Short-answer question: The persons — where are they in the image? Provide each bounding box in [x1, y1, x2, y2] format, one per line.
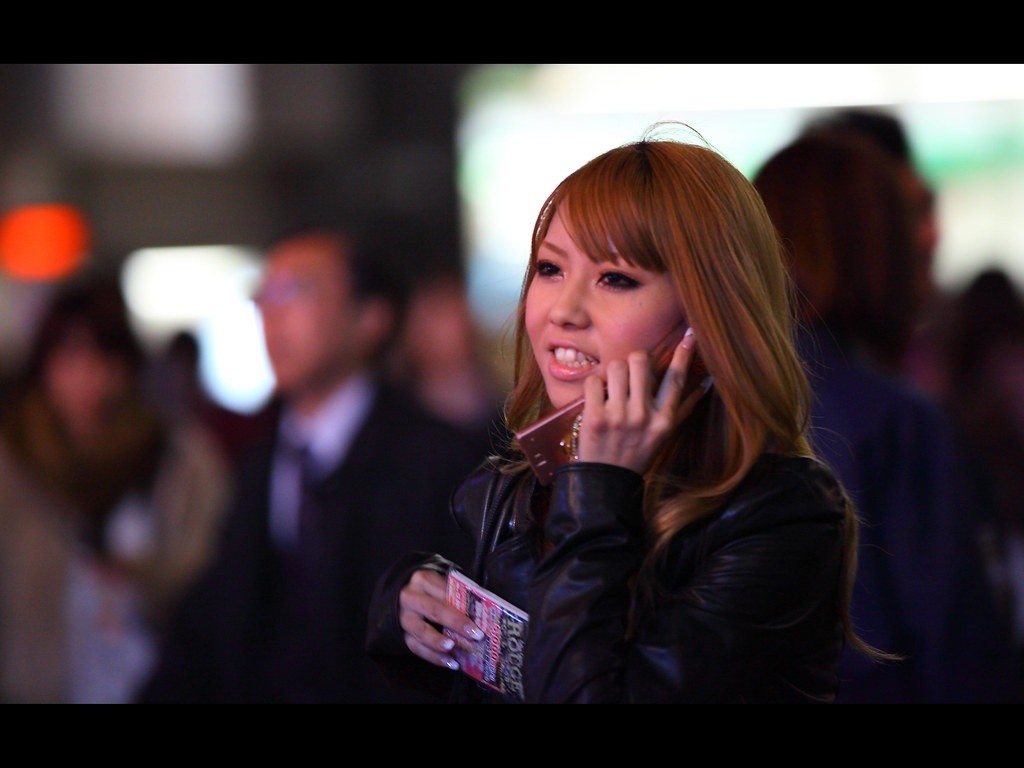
[368, 143, 904, 705]
[753, 107, 1024, 704]
[0, 206, 505, 706]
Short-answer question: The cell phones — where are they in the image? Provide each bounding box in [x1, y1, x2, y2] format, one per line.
[516, 325, 710, 486]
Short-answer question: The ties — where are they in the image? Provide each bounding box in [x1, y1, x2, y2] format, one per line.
[294, 447, 320, 538]
